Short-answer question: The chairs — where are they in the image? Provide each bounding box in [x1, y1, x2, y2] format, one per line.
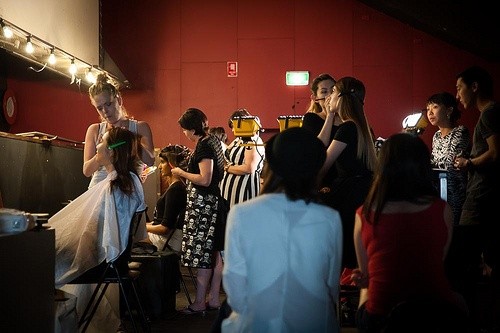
[64, 206, 150, 333]
[162, 212, 197, 306]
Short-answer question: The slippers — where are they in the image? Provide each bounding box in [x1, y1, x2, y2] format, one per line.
[206, 303, 220, 314]
[180, 306, 207, 318]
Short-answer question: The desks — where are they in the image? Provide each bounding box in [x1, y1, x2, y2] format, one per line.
[119, 251, 181, 330]
[0, 228, 55, 333]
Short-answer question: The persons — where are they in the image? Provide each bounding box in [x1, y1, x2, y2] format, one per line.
[316, 76, 380, 272]
[140, 145, 190, 252]
[218, 109, 267, 210]
[302, 72, 342, 207]
[426, 92, 472, 227]
[213, 127, 343, 333]
[169, 106, 228, 318]
[41, 127, 147, 333]
[82, 75, 155, 188]
[442, 65, 500, 333]
[351, 133, 454, 333]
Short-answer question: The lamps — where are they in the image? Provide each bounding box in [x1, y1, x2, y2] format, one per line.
[401, 112, 427, 135]
[233, 115, 264, 141]
[277, 115, 304, 132]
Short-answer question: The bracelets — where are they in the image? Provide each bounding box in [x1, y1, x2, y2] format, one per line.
[466, 159, 473, 168]
